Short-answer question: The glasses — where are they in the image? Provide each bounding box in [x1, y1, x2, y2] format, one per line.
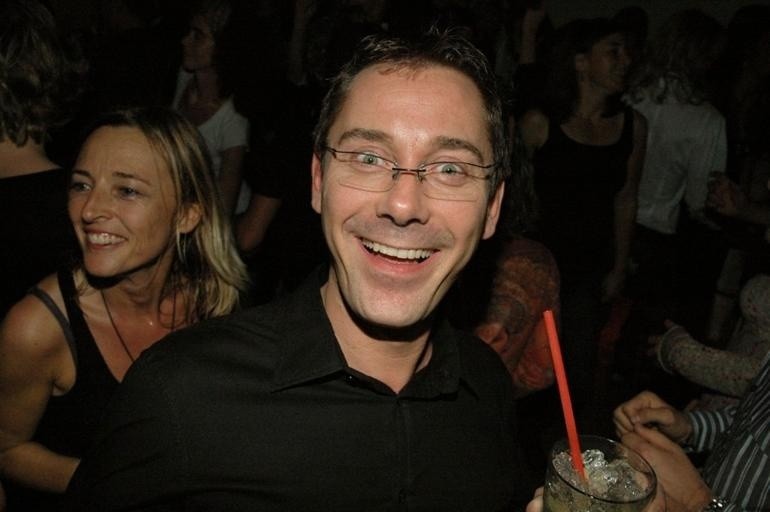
[326, 147, 495, 200]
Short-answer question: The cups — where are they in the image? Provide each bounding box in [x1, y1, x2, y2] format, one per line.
[541, 434, 657, 512]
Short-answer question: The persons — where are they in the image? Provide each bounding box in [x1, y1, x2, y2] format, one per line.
[449, 12, 770, 512]
[48, 25, 538, 511]
[3, 14, 332, 512]
